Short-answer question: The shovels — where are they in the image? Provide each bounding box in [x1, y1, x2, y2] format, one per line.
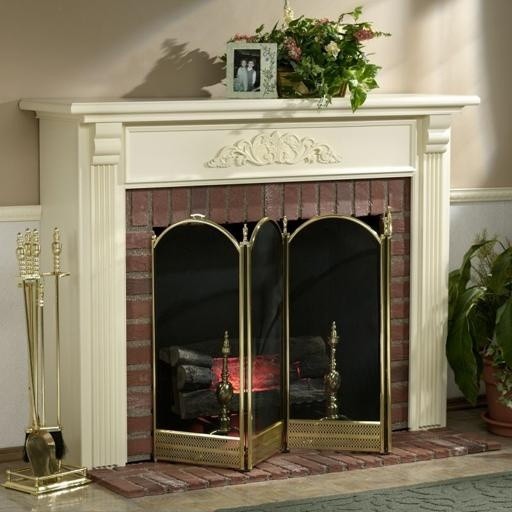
[16, 231, 59, 485]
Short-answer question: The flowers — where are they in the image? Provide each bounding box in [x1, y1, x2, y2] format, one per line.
[219, 0, 392, 116]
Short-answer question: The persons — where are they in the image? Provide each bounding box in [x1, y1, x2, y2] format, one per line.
[237, 59, 248, 92]
[248, 59, 257, 91]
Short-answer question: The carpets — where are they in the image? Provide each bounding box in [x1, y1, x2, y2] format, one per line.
[214, 467, 512, 512]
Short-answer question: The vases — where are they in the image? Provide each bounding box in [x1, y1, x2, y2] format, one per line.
[278, 65, 347, 99]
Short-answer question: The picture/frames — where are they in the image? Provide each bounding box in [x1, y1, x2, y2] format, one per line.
[226, 42, 278, 99]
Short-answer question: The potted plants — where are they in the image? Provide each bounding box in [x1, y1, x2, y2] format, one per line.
[445, 226, 512, 439]
